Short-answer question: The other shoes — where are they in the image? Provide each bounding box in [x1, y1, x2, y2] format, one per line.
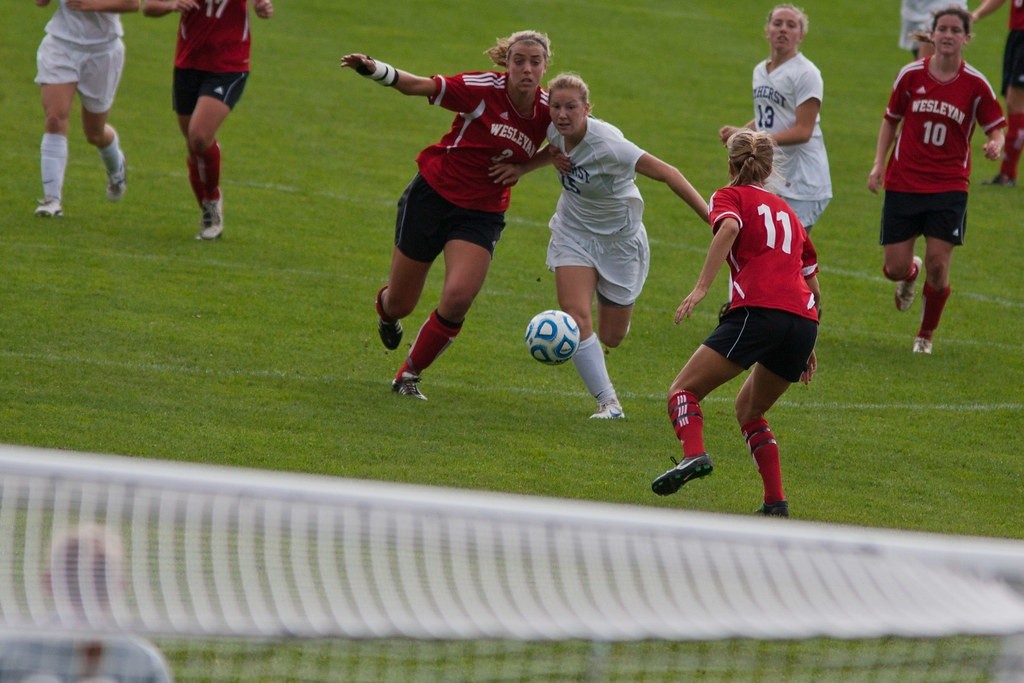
[981, 173, 1013, 186]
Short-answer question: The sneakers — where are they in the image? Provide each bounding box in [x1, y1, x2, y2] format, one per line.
[651, 453, 714, 496]
[105, 161, 126, 199]
[198, 189, 224, 240]
[753, 500, 789, 518]
[912, 335, 933, 355]
[894, 257, 922, 312]
[375, 286, 403, 349]
[589, 402, 624, 420]
[390, 377, 427, 402]
[33, 196, 63, 217]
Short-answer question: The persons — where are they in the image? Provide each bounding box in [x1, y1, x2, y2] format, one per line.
[717, 5, 834, 319]
[899, 0, 968, 62]
[491, 74, 714, 420]
[970, 0, 1024, 186]
[142, 0, 274, 239]
[0, 523, 173, 683]
[651, 133, 822, 522]
[33, 0, 141, 218]
[339, 30, 558, 397]
[868, 7, 1010, 353]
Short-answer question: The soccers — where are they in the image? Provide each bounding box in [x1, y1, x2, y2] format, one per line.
[524, 309, 580, 366]
